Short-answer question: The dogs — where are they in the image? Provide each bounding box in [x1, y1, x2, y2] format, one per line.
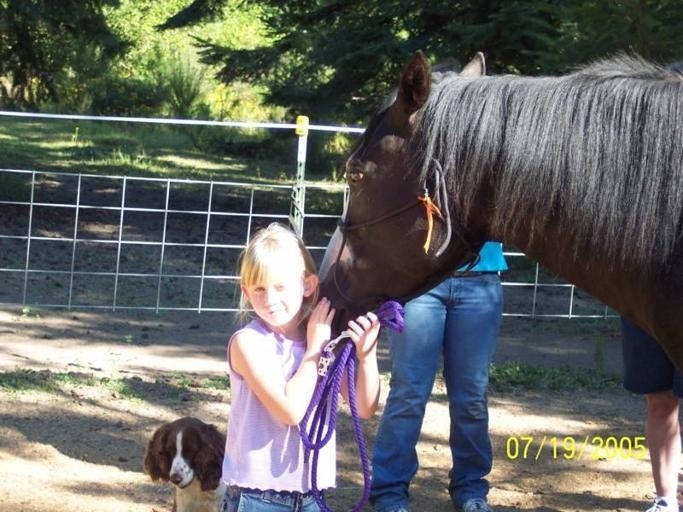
[141, 416, 227, 512]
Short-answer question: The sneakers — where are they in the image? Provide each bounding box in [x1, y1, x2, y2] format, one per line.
[461, 496, 495, 512]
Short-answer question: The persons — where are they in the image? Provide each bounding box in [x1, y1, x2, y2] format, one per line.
[618, 308, 683, 512]
[369, 240, 506, 512]
[219, 221, 381, 511]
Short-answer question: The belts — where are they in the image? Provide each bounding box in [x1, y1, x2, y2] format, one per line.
[452, 271, 501, 277]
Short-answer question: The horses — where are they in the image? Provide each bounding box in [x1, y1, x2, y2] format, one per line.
[304, 51, 683, 374]
